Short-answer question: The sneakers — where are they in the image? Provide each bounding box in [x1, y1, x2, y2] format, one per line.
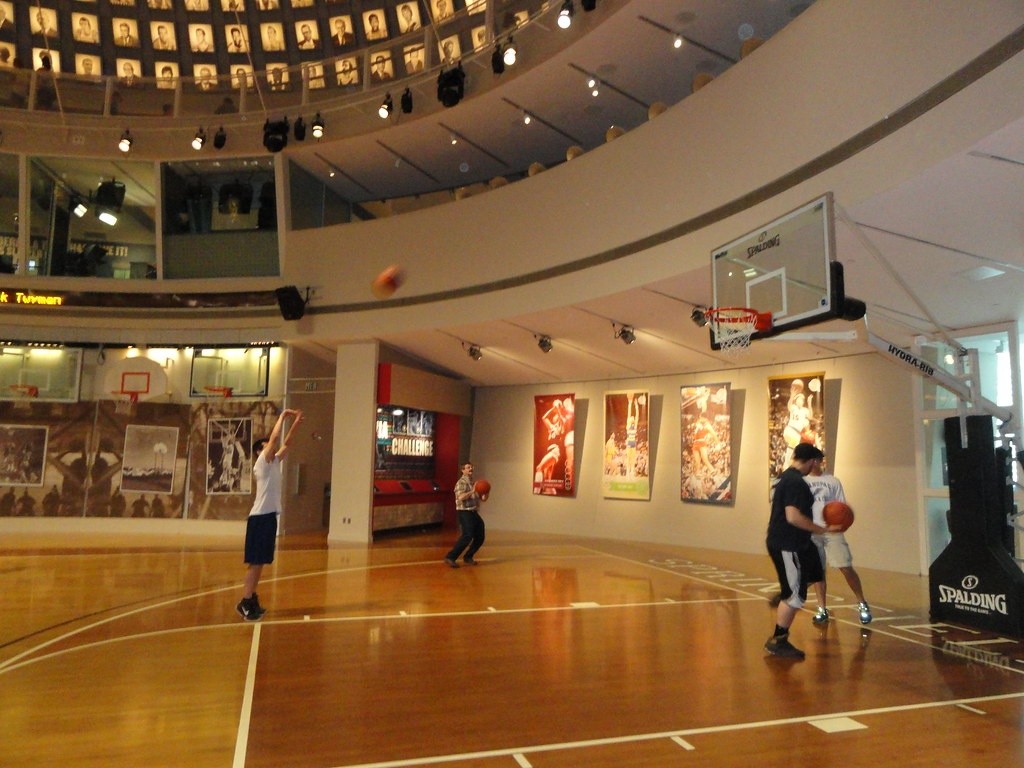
[858, 602, 871, 624]
[235, 599, 260, 621]
[444, 556, 458, 567]
[771, 594, 782, 606]
[251, 594, 266, 615]
[812, 607, 829, 624]
[765, 637, 805, 659]
[463, 556, 478, 566]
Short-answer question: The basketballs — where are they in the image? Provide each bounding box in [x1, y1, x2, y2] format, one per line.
[474, 479, 491, 494]
[822, 500, 854, 533]
[370, 264, 406, 299]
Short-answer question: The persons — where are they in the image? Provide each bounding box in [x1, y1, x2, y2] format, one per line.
[9, 57, 29, 108]
[163, 104, 170, 115]
[110, 91, 123, 115]
[37, 56, 54, 110]
[214, 97, 235, 114]
[765, 442, 843, 657]
[74, 0, 522, 91]
[0, 404, 270, 518]
[770, 386, 826, 479]
[0, 6, 12, 28]
[36, 11, 57, 37]
[605, 398, 648, 477]
[803, 449, 872, 623]
[536, 402, 574, 494]
[0, 47, 11, 66]
[237, 410, 305, 620]
[682, 389, 731, 500]
[445, 461, 489, 567]
[40, 52, 52, 63]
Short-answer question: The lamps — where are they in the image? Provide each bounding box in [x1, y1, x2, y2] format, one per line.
[70, 0, 596, 228]
[690, 305, 708, 327]
[461, 340, 483, 360]
[612, 322, 636, 345]
[534, 334, 553, 354]
